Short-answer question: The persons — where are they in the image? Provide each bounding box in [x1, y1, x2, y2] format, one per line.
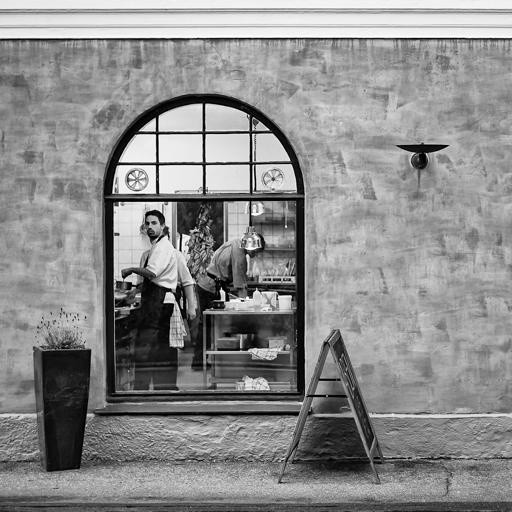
[138, 226, 197, 322]
[122, 210, 178, 390]
[191, 232, 265, 371]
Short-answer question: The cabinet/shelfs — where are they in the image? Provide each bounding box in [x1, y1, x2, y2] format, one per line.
[247, 199, 297, 290]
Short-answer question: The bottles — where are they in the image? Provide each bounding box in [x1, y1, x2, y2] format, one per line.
[253, 287, 262, 311]
[219, 287, 226, 302]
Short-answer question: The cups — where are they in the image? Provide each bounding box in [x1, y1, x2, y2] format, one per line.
[278, 295, 292, 311]
[262, 292, 277, 310]
[211, 300, 224, 309]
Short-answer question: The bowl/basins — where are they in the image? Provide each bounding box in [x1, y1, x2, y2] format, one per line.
[224, 332, 256, 350]
[256, 338, 287, 348]
[230, 299, 253, 311]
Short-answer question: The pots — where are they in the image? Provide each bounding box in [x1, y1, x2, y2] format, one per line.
[116, 281, 137, 290]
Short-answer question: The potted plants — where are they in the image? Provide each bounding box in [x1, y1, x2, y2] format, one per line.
[32, 307, 91, 471]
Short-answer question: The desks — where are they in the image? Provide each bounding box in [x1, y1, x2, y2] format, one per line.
[202, 308, 298, 393]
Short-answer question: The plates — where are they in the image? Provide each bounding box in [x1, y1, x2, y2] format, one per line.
[209, 309, 227, 311]
